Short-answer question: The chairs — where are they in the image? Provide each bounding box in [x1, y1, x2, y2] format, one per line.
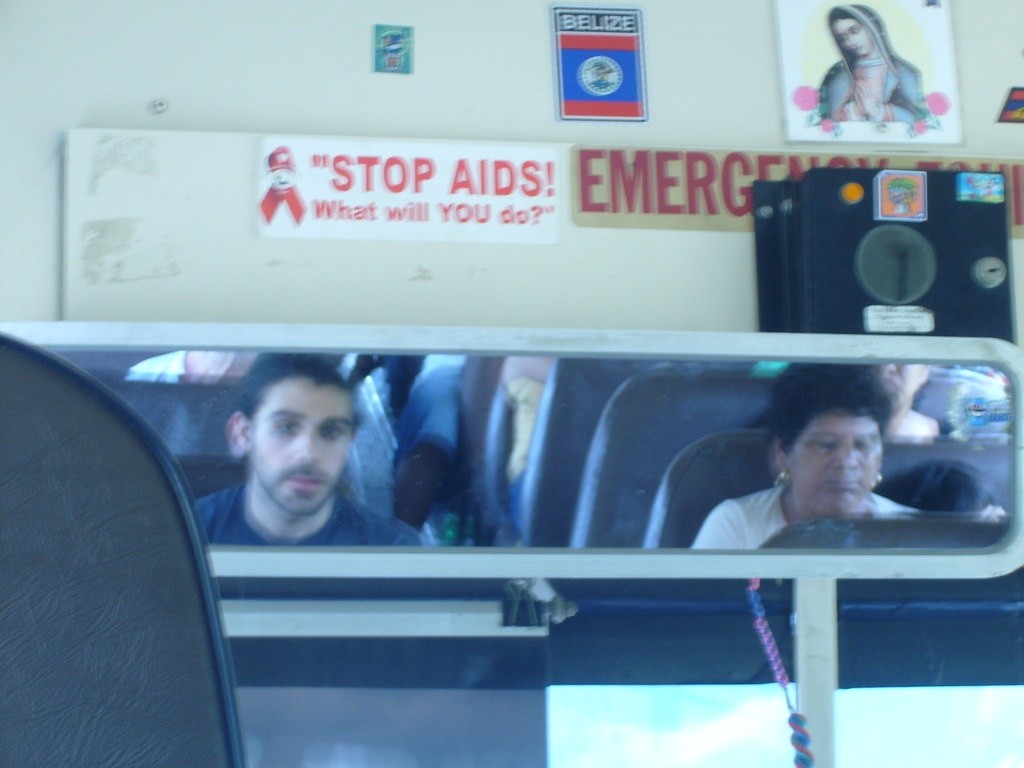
[112, 353, 1014, 547]
[0, 333, 245, 768]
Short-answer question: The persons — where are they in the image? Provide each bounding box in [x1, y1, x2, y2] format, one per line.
[691, 365, 968, 550]
[124, 349, 557, 545]
